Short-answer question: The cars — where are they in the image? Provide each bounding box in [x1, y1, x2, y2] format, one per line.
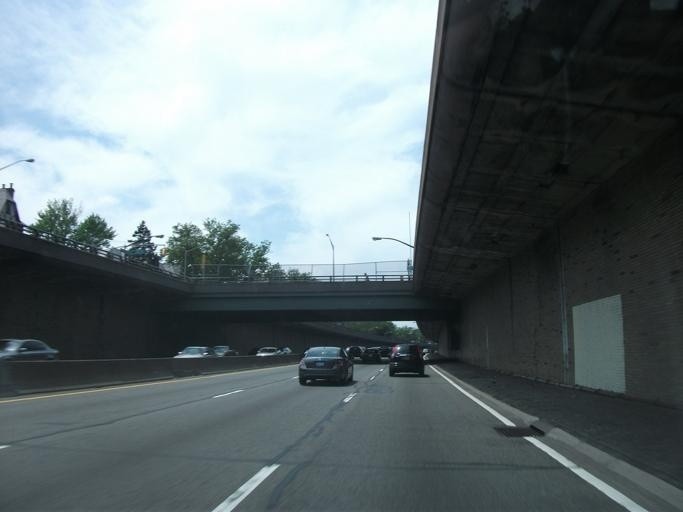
[346, 346, 390, 362]
[1, 338, 62, 360]
[174, 345, 238, 358]
[298, 347, 354, 385]
[390, 344, 425, 376]
[256, 347, 282, 356]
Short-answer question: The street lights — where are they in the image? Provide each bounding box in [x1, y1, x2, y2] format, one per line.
[373, 236, 413, 247]
[1, 158, 33, 172]
[124, 234, 163, 263]
[327, 234, 334, 282]
